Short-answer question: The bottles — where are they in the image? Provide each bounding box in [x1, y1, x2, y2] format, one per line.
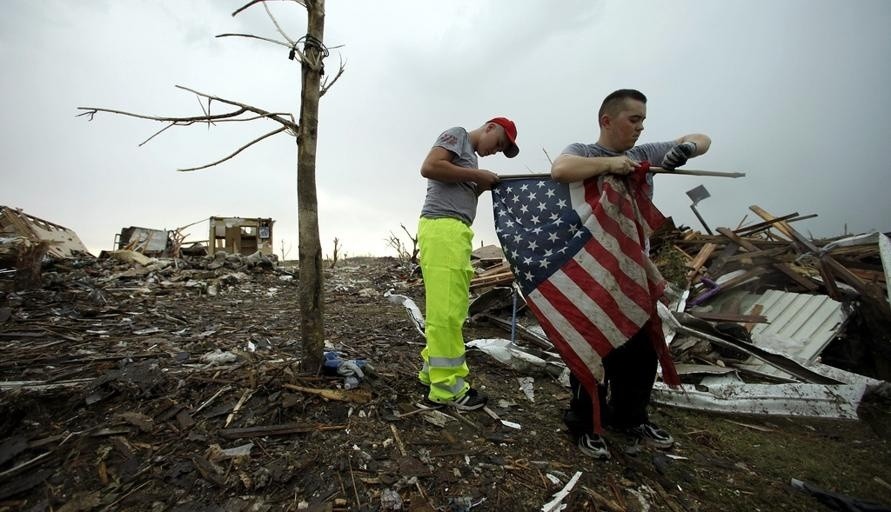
[344, 366, 366, 390]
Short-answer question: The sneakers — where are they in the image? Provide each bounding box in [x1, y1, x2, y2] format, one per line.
[431, 388, 488, 410]
[578, 430, 612, 461]
[633, 423, 674, 449]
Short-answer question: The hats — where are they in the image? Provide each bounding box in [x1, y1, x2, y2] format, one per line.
[487, 118, 519, 157]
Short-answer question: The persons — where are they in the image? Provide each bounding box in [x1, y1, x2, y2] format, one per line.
[550, 88, 713, 461]
[418, 117, 520, 412]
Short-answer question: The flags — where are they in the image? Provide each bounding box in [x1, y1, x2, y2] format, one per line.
[491, 163, 691, 434]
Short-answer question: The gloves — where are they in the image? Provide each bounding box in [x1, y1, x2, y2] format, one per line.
[661, 142, 697, 171]
